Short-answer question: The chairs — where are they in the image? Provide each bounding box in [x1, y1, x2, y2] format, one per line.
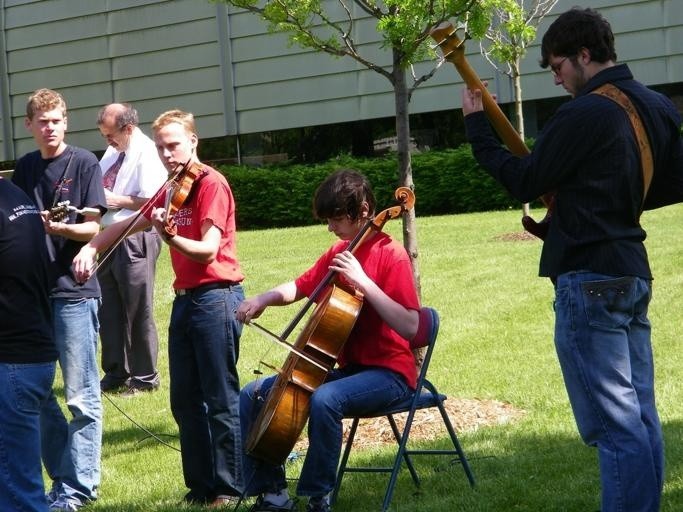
[330, 308, 475, 508]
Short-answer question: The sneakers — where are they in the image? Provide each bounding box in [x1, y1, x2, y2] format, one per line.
[47, 487, 60, 502]
[306, 496, 331, 512]
[250, 493, 299, 511]
[48, 497, 82, 511]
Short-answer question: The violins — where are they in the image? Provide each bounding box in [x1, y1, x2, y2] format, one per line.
[161, 158, 203, 241]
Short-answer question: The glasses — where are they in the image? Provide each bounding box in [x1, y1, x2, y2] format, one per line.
[100, 121, 132, 139]
[550, 56, 569, 76]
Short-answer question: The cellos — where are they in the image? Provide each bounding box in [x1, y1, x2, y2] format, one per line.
[243, 187, 415, 464]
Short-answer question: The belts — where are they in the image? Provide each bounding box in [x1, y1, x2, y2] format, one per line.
[174, 279, 240, 296]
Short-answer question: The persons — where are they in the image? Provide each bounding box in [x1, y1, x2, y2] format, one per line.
[461, 8, 683, 511]
[97, 102, 169, 394]
[235, 168, 422, 512]
[11, 89, 104, 511]
[0, 176, 58, 512]
[71, 109, 246, 507]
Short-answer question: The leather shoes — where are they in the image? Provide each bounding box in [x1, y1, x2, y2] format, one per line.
[179, 494, 209, 506]
[122, 384, 159, 394]
[100, 377, 127, 391]
[209, 496, 231, 509]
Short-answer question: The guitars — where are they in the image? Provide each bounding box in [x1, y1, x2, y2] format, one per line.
[427, 21, 561, 240]
[46, 203, 99, 222]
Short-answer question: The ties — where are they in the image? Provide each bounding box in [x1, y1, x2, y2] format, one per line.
[103, 152, 126, 191]
